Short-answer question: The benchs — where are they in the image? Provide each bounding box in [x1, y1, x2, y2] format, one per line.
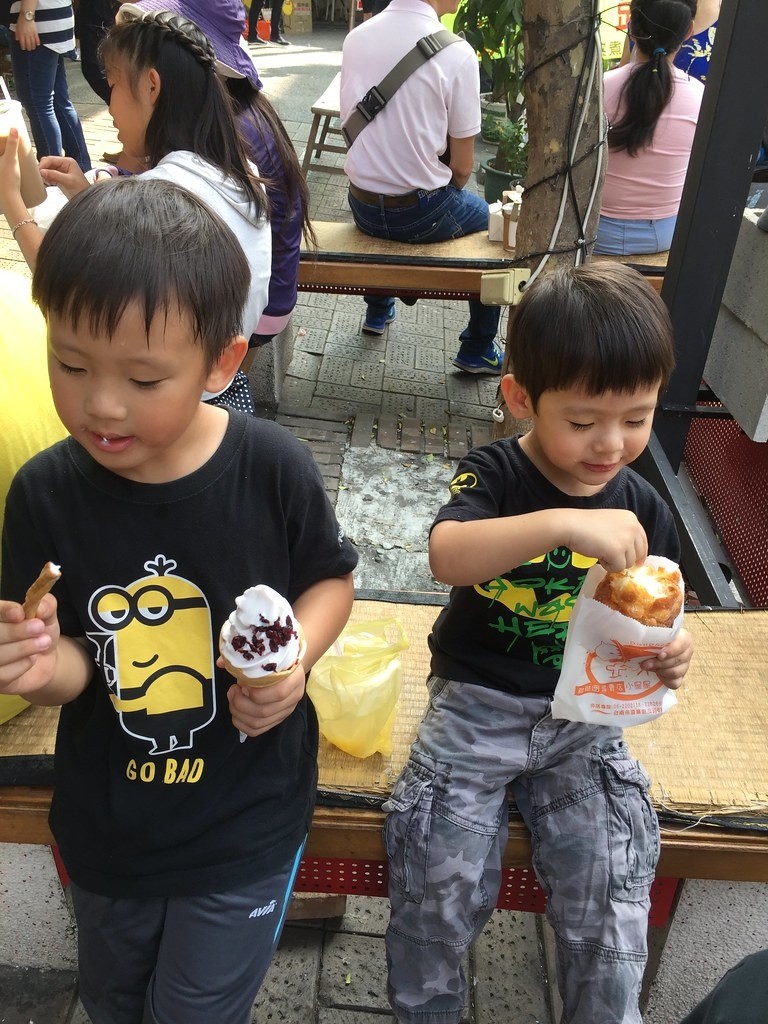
[296, 221, 671, 303]
[0, 588, 768, 883]
[301, 71, 349, 182]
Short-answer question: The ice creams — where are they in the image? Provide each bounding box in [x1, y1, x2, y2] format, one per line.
[217, 581, 307, 744]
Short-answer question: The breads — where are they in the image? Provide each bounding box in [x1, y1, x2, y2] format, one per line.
[596, 565, 684, 628]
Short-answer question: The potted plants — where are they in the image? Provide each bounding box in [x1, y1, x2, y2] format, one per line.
[453, 0, 528, 204]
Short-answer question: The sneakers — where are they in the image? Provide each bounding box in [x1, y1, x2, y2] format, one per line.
[362, 304, 396, 335]
[452, 342, 506, 375]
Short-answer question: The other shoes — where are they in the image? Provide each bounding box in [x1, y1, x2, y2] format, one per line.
[269, 37, 290, 45]
[248, 36, 268, 45]
[75, 47, 81, 61]
[103, 143, 123, 163]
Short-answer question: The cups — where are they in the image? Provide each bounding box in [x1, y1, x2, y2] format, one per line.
[502, 204, 522, 252]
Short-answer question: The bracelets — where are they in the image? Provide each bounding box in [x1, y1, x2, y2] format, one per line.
[12, 219, 38, 240]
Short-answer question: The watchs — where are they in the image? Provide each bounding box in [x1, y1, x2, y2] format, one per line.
[19, 10, 36, 21]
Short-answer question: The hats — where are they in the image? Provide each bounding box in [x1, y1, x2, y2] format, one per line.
[118, 0, 264, 92]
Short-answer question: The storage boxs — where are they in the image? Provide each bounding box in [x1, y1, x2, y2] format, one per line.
[242, 20, 271, 41]
[281, 0, 312, 35]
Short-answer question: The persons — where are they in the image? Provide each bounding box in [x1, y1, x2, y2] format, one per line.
[339, 0, 505, 373]
[593, 1, 704, 258]
[0, 183, 356, 1023]
[0, 12, 273, 402]
[246, 1, 293, 47]
[0, 0, 91, 186]
[384, 260, 695, 1022]
[94, 1, 322, 349]
[619, 1, 720, 83]
